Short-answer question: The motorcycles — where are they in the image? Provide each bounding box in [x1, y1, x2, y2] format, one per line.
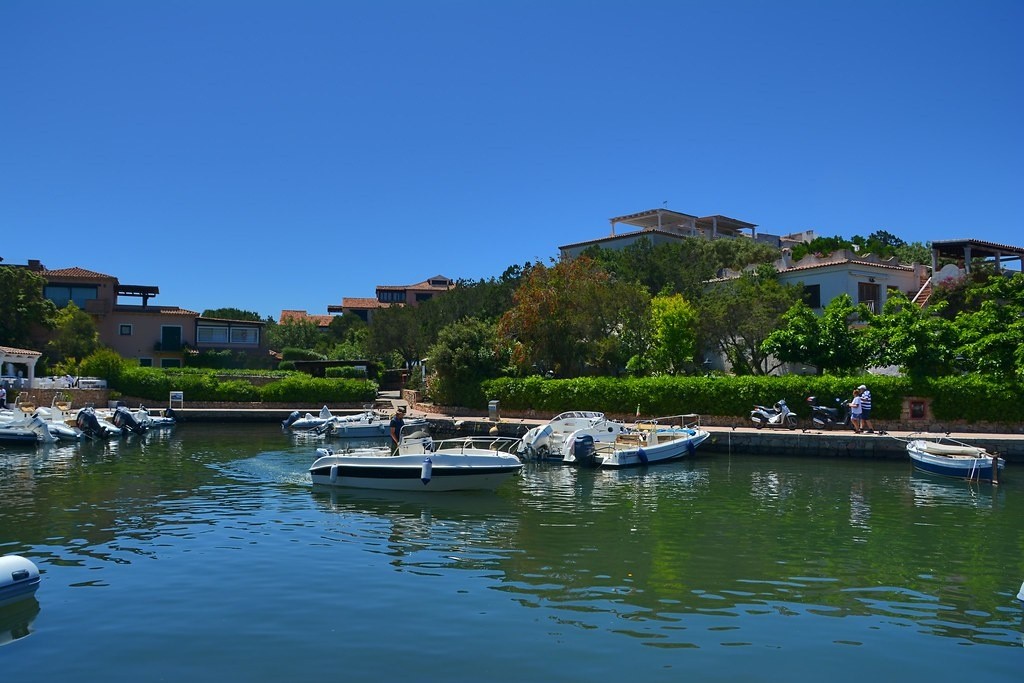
[807, 395, 861, 430]
[750, 397, 798, 431]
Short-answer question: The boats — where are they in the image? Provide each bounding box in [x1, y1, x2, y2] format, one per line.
[308, 431, 524, 490]
[574, 412, 710, 468]
[291, 404, 432, 439]
[517, 411, 611, 463]
[907, 439, 1009, 480]
[0, 391, 176, 442]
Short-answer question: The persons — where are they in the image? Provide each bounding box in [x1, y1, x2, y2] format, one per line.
[857, 385, 874, 433]
[0, 385, 10, 409]
[848, 390, 862, 434]
[390, 407, 406, 456]
[64, 374, 75, 388]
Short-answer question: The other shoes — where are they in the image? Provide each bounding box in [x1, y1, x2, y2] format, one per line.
[853, 431, 863, 434]
[865, 430, 873, 434]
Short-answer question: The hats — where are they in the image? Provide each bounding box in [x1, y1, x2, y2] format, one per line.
[858, 385, 866, 390]
